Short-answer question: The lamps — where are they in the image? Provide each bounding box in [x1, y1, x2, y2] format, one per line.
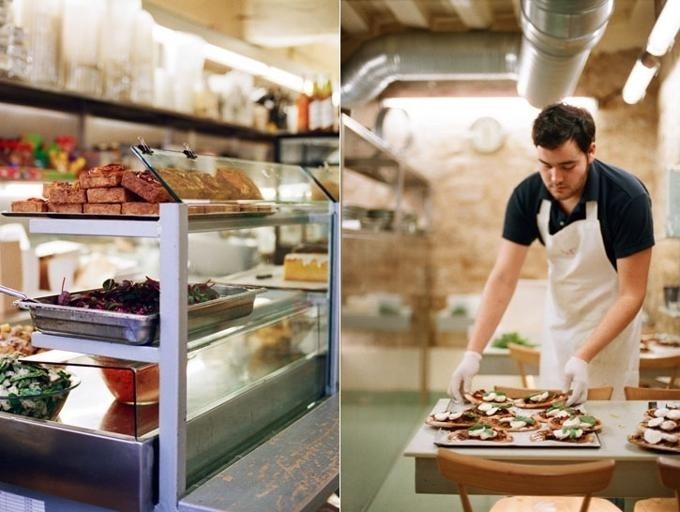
[622, 0, 679, 106]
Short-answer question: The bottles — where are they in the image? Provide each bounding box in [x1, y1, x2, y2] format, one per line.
[253, 80, 335, 139]
[2, 136, 123, 180]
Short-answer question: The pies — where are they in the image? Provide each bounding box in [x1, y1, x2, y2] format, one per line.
[425, 390, 680, 453]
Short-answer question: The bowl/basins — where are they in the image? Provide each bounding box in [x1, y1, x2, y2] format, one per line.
[1, 375, 81, 423]
[88, 354, 160, 407]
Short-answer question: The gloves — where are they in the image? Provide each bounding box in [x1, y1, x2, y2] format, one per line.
[448, 350, 483, 401]
[560, 355, 591, 408]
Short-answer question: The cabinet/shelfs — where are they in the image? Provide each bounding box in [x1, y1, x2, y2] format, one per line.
[0, 197, 339, 512]
[269, 128, 340, 260]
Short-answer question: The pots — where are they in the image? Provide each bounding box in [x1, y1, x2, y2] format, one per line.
[340, 204, 415, 232]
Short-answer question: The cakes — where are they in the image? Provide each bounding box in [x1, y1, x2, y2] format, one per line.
[283, 243, 329, 282]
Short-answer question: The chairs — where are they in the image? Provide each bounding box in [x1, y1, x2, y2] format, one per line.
[631, 456, 680, 512]
[435, 445, 624, 512]
[490, 331, 680, 401]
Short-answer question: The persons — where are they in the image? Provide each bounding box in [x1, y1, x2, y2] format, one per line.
[445, 102, 658, 410]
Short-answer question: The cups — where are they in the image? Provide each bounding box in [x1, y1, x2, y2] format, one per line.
[0, 3, 160, 109]
[663, 287, 680, 312]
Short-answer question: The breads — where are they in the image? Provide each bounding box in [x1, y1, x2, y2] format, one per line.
[12, 164, 271, 217]
